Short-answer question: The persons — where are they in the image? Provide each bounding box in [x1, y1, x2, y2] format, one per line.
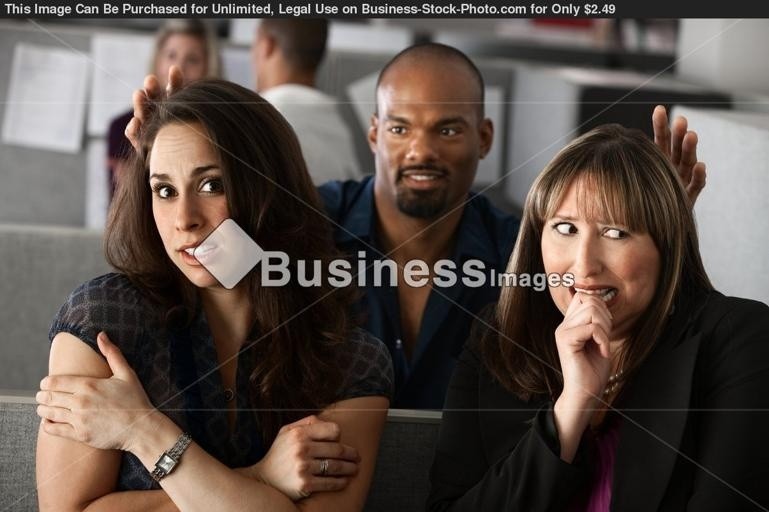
[36, 18, 768, 512]
